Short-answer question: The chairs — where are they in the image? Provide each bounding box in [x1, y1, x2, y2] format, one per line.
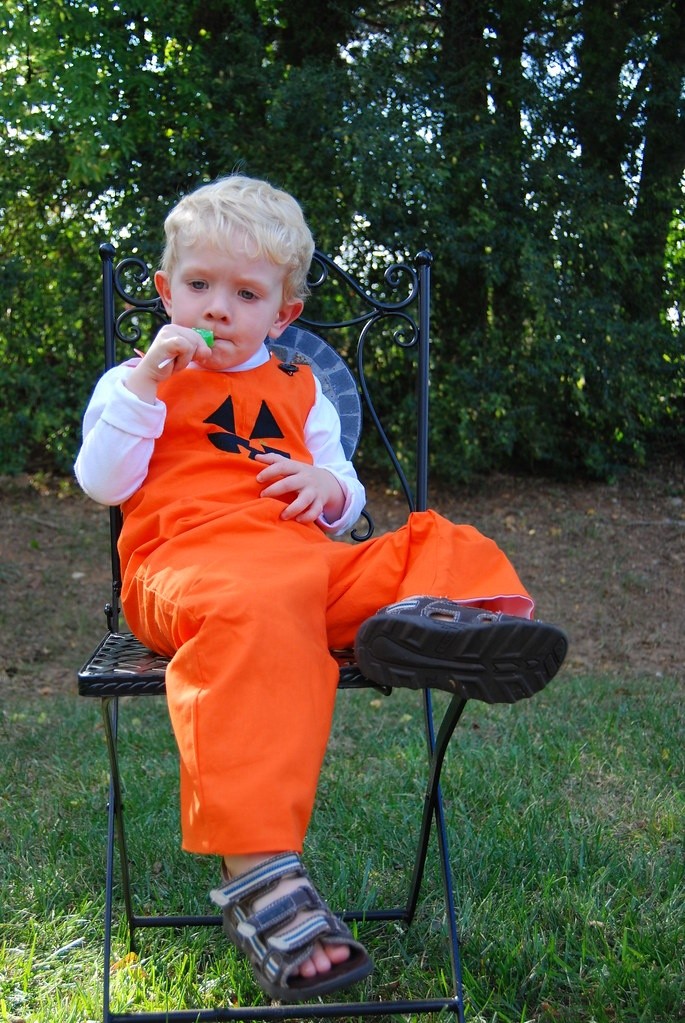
[78, 243, 468, 1023]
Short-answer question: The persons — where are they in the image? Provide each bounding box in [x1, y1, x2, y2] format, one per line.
[65, 176, 572, 1006]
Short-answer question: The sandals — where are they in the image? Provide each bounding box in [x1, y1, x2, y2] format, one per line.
[209, 849, 374, 1003]
[354, 594, 568, 704]
